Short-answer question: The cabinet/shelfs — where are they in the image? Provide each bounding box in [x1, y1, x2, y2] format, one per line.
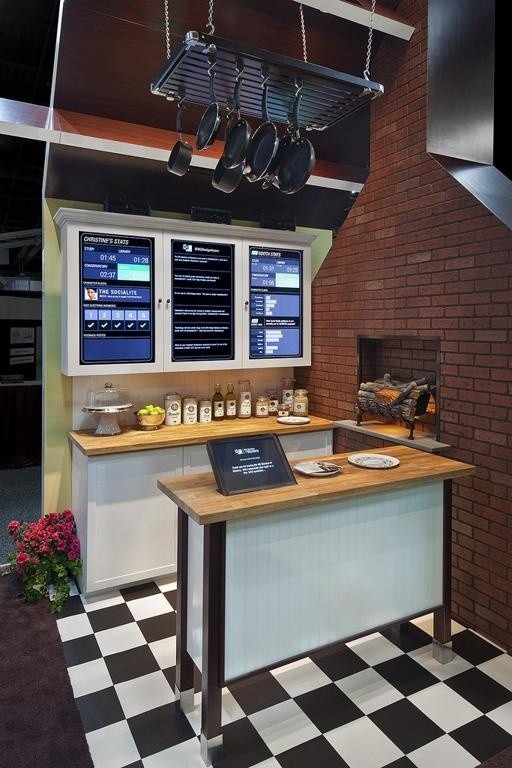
[68, 431, 214, 596]
[243, 227, 317, 368]
[54, 208, 243, 377]
[190, 423, 335, 475]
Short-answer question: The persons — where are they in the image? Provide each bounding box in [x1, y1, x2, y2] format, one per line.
[86, 287, 97, 301]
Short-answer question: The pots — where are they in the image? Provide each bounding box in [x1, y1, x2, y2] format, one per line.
[167, 51, 315, 198]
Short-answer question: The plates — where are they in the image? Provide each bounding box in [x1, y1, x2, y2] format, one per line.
[347, 453, 400, 470]
[296, 460, 342, 478]
[275, 415, 311, 424]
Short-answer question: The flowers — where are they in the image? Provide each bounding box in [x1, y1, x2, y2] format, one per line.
[7, 509, 81, 615]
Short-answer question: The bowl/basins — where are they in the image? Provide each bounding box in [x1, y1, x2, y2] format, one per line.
[133, 411, 165, 430]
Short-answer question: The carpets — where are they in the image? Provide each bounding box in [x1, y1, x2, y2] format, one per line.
[0, 596, 94, 767]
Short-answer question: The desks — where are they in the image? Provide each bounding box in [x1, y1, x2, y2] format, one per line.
[157, 445, 477, 766]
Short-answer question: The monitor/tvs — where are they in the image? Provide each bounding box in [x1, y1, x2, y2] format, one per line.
[205, 432, 297, 497]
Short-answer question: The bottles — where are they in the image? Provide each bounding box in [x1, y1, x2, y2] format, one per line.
[237, 378, 252, 420]
[227, 383, 238, 420]
[255, 375, 308, 418]
[212, 383, 225, 420]
